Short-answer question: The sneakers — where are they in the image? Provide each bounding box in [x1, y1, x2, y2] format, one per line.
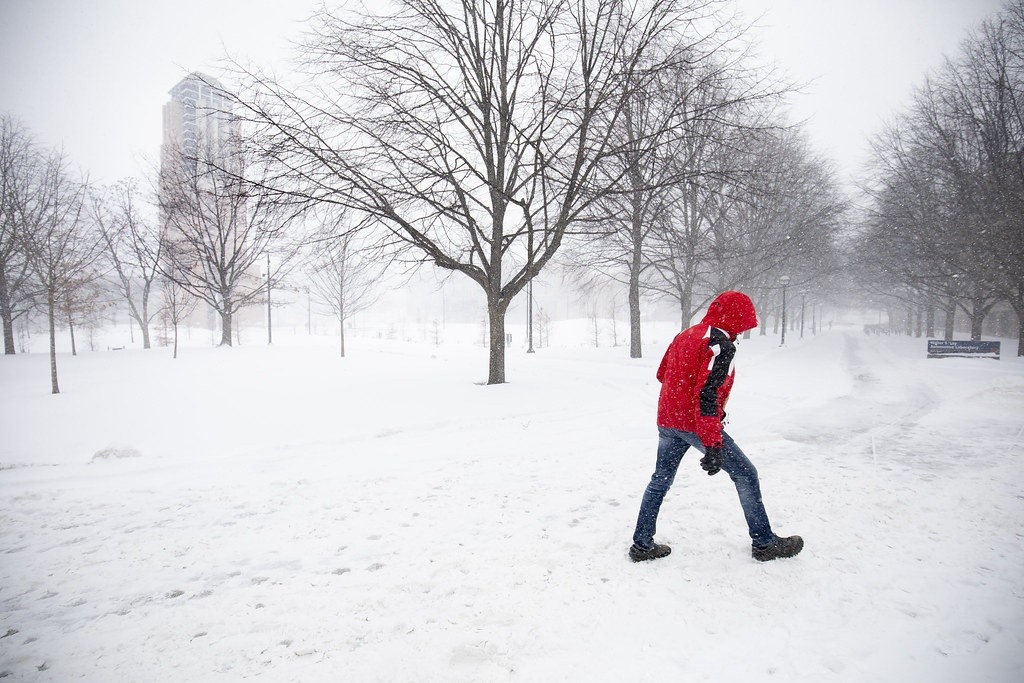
[752, 535, 803, 562]
[630, 545, 670, 561]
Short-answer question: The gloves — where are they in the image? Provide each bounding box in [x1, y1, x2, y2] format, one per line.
[700, 447, 722, 475]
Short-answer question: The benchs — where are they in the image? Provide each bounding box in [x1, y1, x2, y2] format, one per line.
[926, 340, 1001, 359]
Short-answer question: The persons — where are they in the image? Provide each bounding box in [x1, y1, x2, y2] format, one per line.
[629, 290, 804, 560]
